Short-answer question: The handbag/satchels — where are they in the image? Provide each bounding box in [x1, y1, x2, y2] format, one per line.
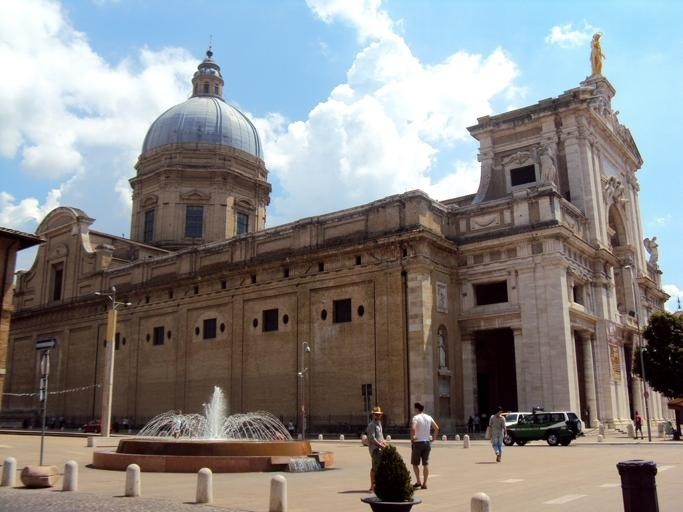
[484, 426, 492, 440]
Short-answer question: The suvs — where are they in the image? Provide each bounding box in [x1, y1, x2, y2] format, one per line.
[503, 408, 581, 445]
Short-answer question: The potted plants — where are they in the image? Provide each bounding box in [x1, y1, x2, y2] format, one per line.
[361, 441, 424, 511]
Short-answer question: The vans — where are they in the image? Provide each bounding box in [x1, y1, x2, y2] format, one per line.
[500, 412, 532, 427]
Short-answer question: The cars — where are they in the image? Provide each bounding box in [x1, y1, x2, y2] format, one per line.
[81, 420, 113, 433]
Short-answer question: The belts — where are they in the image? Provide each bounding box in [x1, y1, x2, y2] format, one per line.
[413, 441, 430, 444]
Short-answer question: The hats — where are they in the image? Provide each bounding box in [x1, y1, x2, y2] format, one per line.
[371, 406, 383, 415]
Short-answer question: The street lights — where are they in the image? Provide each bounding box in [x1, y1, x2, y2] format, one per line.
[623, 265, 652, 442]
[94, 286, 133, 436]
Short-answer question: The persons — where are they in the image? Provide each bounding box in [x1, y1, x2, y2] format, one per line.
[28, 416, 66, 431]
[109, 415, 137, 434]
[365, 405, 390, 490]
[437, 328, 449, 369]
[408, 401, 439, 489]
[467, 415, 473, 433]
[603, 175, 629, 207]
[648, 235, 660, 263]
[473, 413, 480, 433]
[589, 32, 607, 74]
[287, 418, 295, 438]
[480, 414, 487, 431]
[489, 406, 507, 461]
[633, 410, 643, 439]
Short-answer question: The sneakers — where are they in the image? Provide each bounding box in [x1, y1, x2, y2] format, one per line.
[421, 485, 427, 489]
[413, 482, 421, 488]
[496, 454, 501, 462]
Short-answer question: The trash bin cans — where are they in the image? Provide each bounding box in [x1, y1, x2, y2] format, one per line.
[617, 460, 659, 512]
